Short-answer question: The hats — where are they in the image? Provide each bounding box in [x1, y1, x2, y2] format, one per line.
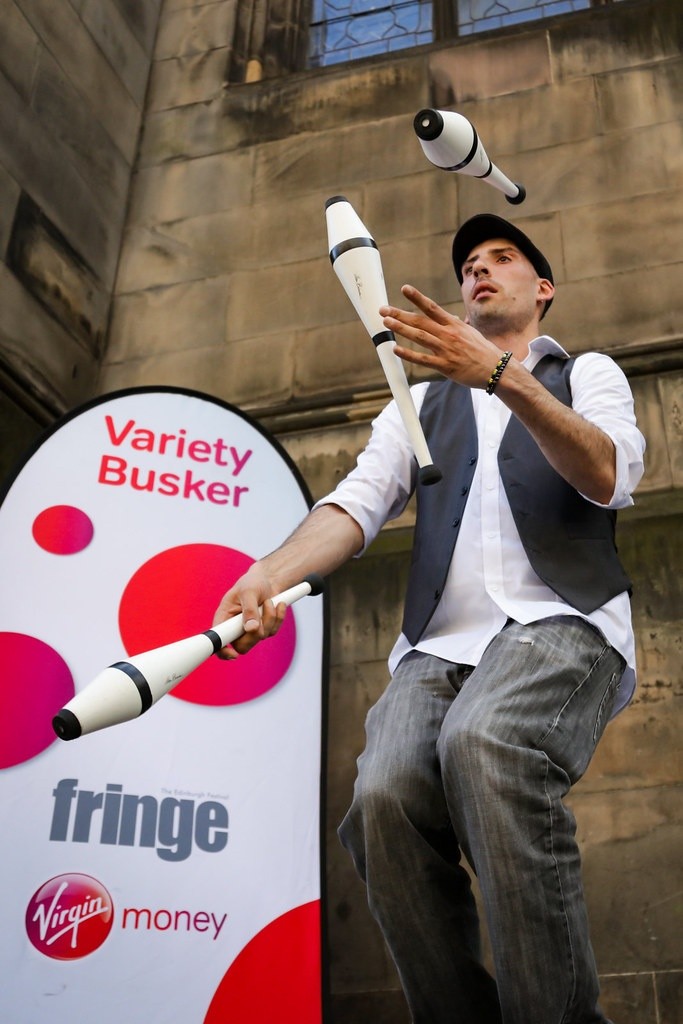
[452, 213, 555, 321]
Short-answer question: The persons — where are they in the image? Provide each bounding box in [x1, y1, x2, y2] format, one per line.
[212, 213, 646, 1024]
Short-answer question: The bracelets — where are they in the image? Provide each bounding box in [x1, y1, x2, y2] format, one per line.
[486, 350, 514, 396]
